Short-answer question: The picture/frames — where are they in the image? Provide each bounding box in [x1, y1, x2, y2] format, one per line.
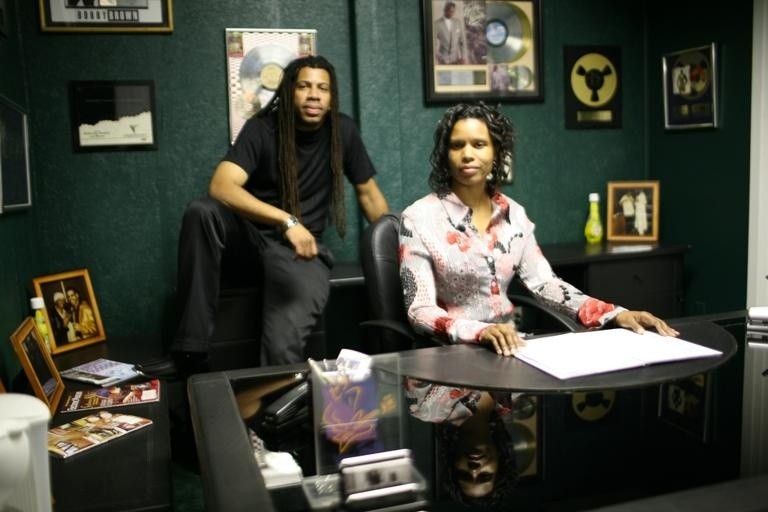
[12, 318, 65, 415]
[662, 44, 717, 129]
[0, 114, 34, 214]
[418, 0, 545, 107]
[71, 81, 156, 149]
[32, 270, 105, 354]
[41, 1, 176, 32]
[606, 181, 659, 242]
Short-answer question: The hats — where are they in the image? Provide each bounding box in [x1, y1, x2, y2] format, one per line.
[53, 292, 64, 302]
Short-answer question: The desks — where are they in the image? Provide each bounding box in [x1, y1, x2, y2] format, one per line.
[10, 334, 173, 512]
[186, 314, 768, 512]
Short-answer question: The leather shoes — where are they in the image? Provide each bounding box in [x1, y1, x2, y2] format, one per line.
[137, 351, 219, 380]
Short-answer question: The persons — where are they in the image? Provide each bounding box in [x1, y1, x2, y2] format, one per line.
[676, 69, 688, 94]
[435, 2, 468, 65]
[50, 292, 80, 347]
[402, 373, 517, 509]
[67, 288, 98, 339]
[634, 189, 647, 237]
[166, 56, 391, 365]
[396, 104, 680, 359]
[491, 65, 509, 92]
[620, 189, 635, 235]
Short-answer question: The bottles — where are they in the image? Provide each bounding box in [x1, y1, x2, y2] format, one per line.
[584, 193, 604, 243]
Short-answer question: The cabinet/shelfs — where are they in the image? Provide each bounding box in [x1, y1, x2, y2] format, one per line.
[323, 240, 688, 355]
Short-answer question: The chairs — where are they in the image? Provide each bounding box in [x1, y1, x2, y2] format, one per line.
[350, 212, 589, 352]
